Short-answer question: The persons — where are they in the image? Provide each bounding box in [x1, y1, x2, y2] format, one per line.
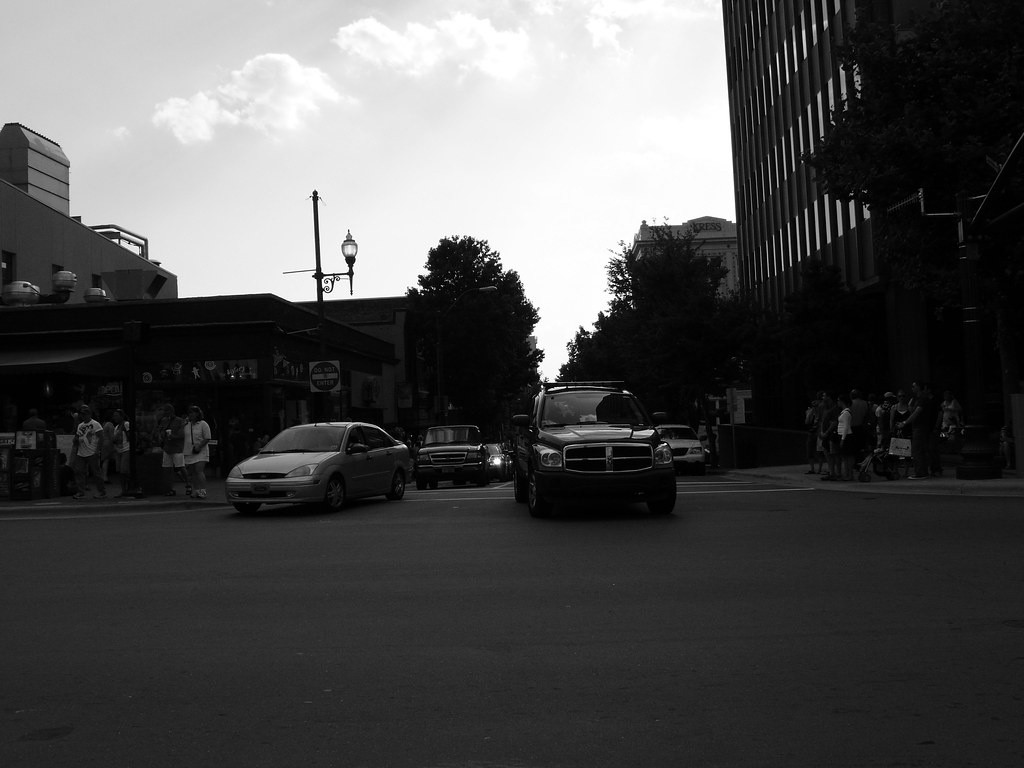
[2, 402, 213, 501]
[807, 382, 962, 480]
[224, 415, 423, 473]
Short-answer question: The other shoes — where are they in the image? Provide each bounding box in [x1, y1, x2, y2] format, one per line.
[804, 470, 816, 474]
[113, 492, 133, 498]
[93, 492, 107, 498]
[820, 474, 837, 480]
[73, 493, 85, 499]
[842, 475, 854, 481]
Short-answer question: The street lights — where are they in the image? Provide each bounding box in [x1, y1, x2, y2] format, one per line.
[283, 191, 358, 422]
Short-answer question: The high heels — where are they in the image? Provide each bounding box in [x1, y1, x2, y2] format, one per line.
[165, 490, 177, 496]
[185, 485, 192, 495]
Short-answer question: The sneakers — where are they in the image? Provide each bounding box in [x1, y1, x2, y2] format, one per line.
[908, 473, 928, 480]
[190, 489, 208, 498]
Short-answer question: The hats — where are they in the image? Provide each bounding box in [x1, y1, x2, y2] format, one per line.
[884, 392, 896, 397]
[80, 408, 92, 415]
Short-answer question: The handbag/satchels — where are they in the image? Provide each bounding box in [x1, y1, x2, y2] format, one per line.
[191, 422, 199, 454]
[160, 420, 184, 455]
[888, 430, 911, 457]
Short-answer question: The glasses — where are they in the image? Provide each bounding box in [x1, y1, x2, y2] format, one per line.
[898, 395, 905, 398]
[912, 385, 915, 388]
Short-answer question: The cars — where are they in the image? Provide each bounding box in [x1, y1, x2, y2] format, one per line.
[510, 381, 676, 518]
[416, 425, 705, 490]
[226, 422, 410, 512]
